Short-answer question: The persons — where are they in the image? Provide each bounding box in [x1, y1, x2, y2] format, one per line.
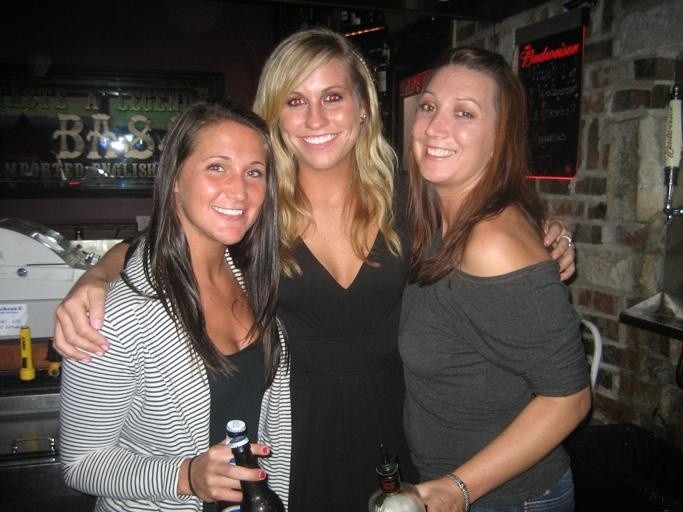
[52, 28, 576, 512]
[58, 104, 291, 511]
[398, 45, 593, 511]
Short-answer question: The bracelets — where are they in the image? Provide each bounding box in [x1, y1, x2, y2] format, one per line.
[446, 472, 470, 512]
[187, 455, 199, 497]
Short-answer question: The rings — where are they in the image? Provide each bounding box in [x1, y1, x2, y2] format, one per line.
[562, 235, 573, 247]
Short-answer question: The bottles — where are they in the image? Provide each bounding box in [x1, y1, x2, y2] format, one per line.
[208, 421, 287, 512]
[365, 442, 425, 512]
